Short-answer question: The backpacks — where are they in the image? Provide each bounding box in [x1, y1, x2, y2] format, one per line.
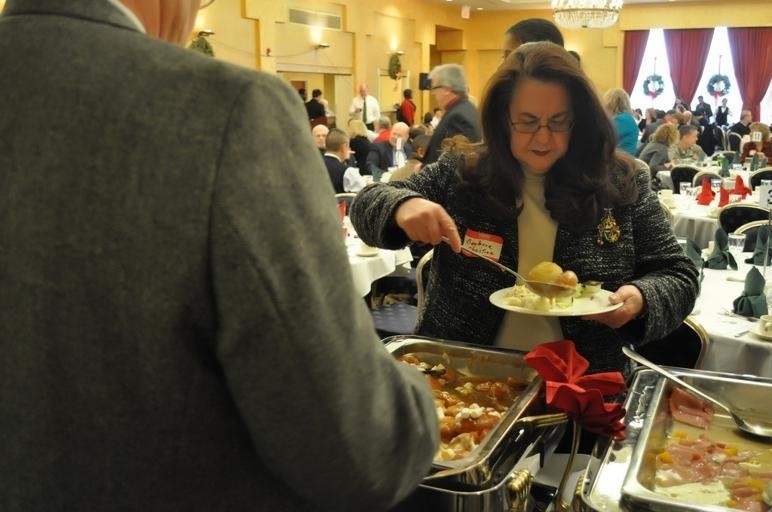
[396, 106, 402, 122]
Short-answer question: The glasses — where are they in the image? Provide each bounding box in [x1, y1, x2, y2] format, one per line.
[505, 109, 574, 132]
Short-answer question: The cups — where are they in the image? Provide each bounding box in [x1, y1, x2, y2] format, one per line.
[758, 316, 771, 334]
[728, 233, 746, 262]
[710, 178, 721, 195]
[733, 162, 744, 177]
[679, 181, 691, 194]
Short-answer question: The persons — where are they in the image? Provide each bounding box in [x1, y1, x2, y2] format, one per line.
[635, 108, 700, 128]
[420, 63, 482, 170]
[501, 18, 581, 62]
[716, 99, 732, 126]
[0, 0, 440, 512]
[674, 98, 688, 109]
[351, 41, 700, 373]
[638, 123, 680, 179]
[602, 88, 639, 156]
[389, 134, 434, 182]
[348, 120, 372, 175]
[467, 87, 481, 110]
[730, 111, 753, 151]
[431, 108, 445, 130]
[697, 96, 713, 123]
[348, 82, 380, 132]
[298, 88, 335, 118]
[372, 116, 392, 143]
[312, 125, 330, 160]
[423, 112, 433, 128]
[404, 124, 429, 160]
[366, 122, 411, 182]
[401, 89, 416, 126]
[324, 128, 348, 193]
[671, 125, 708, 161]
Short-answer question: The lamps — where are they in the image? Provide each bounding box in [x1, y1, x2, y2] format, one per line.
[320, 43, 330, 47]
[552, 0, 623, 30]
[198, 27, 213, 36]
[398, 50, 404, 55]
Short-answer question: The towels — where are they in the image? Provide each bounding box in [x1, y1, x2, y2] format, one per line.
[745, 224, 772, 267]
[696, 176, 715, 205]
[733, 173, 753, 199]
[707, 227, 738, 270]
[719, 185, 730, 207]
[733, 266, 769, 319]
[686, 236, 704, 269]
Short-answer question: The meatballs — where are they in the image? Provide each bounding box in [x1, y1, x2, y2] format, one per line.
[559, 269, 578, 288]
[526, 260, 563, 295]
[489, 381, 511, 401]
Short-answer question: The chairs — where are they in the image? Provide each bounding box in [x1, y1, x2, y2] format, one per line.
[692, 170, 722, 189]
[334, 192, 357, 216]
[369, 248, 434, 341]
[671, 164, 701, 193]
[736, 220, 772, 252]
[748, 167, 772, 191]
[718, 202, 772, 234]
[636, 316, 709, 368]
[371, 267, 419, 306]
[713, 151, 737, 164]
[717, 126, 726, 151]
[728, 131, 741, 150]
[722, 124, 730, 150]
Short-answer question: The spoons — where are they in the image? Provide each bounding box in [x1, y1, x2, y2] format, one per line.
[618, 346, 772, 442]
[441, 236, 577, 298]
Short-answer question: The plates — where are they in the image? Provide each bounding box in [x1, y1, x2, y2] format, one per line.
[385, 338, 543, 485]
[572, 367, 772, 510]
[380, 334, 568, 511]
[622, 369, 770, 511]
[748, 324, 772, 338]
[490, 285, 623, 314]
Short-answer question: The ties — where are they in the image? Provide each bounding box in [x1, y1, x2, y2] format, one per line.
[363, 98, 367, 123]
